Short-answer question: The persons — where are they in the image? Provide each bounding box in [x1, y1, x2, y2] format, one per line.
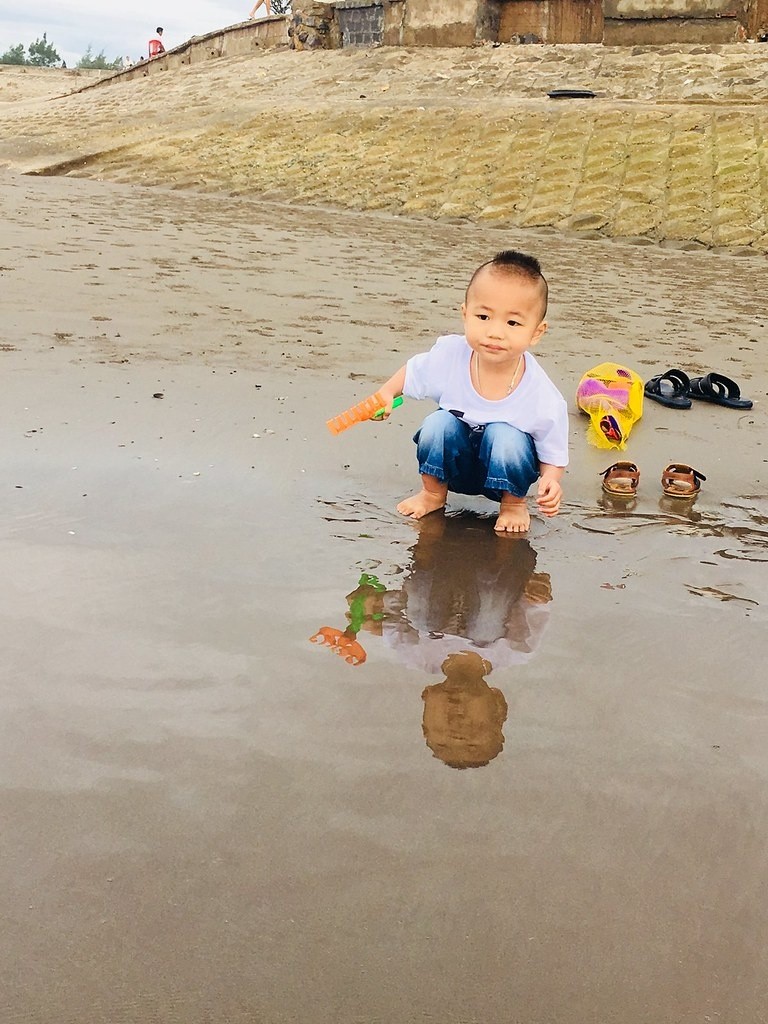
[140, 57, 143, 60]
[357, 250, 568, 533]
[149, 27, 164, 55]
[124, 57, 134, 69]
[249, 0, 271, 19]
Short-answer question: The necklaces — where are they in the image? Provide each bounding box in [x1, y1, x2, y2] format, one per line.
[475, 353, 524, 398]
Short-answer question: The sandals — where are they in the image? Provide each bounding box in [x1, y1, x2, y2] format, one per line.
[661, 463, 708, 499]
[599, 460, 641, 497]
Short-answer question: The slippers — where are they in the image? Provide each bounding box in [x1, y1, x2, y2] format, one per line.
[246, 17, 254, 20]
[689, 372, 753, 409]
[644, 369, 692, 409]
